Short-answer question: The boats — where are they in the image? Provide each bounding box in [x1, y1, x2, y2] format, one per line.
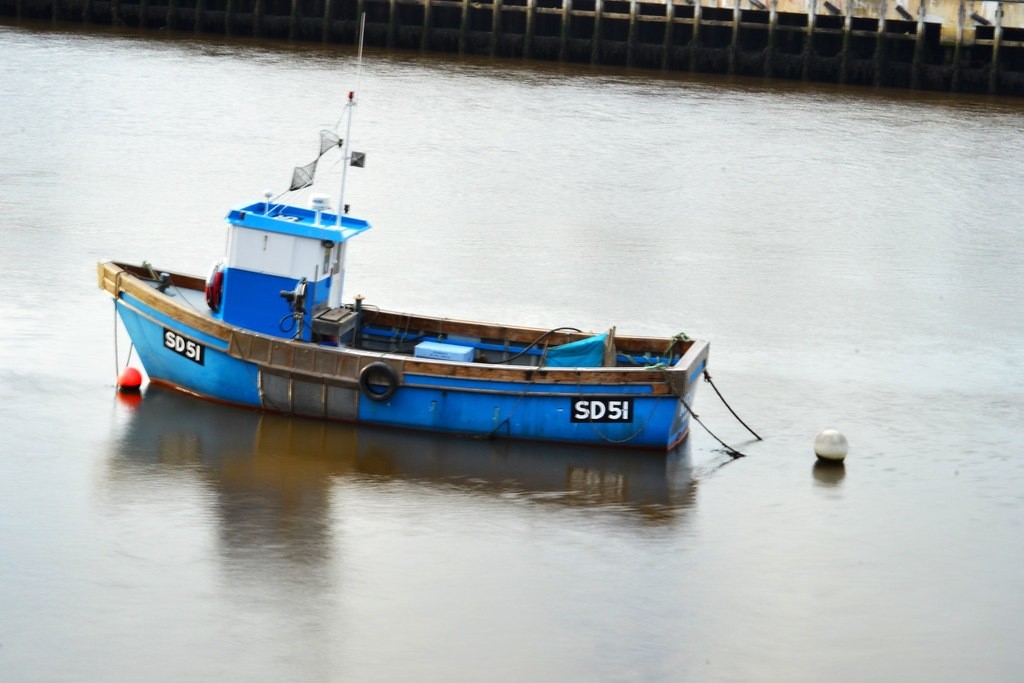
[97, 10, 717, 450]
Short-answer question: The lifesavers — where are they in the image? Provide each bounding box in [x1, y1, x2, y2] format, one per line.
[205, 260, 226, 312]
[358, 361, 398, 402]
